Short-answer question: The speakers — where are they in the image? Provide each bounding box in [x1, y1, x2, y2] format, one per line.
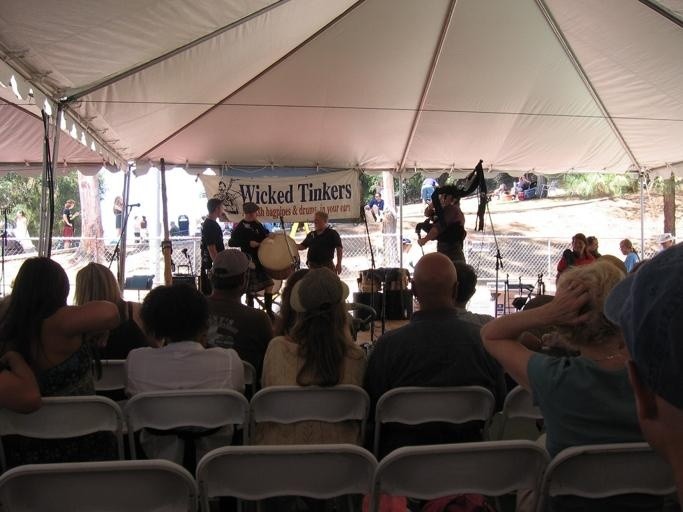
[524, 295, 555, 310]
[174, 274, 193, 286]
[353, 291, 412, 321]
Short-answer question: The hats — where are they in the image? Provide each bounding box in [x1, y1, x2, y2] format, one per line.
[288, 266, 350, 313]
[603, 241, 683, 411]
[242, 201, 260, 214]
[207, 197, 225, 213]
[211, 248, 252, 278]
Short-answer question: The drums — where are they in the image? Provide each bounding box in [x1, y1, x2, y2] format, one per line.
[259, 234, 300, 279]
[252, 278, 282, 295]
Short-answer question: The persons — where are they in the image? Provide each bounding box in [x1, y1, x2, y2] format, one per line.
[291, 212, 342, 274]
[495, 179, 507, 194]
[63, 198, 80, 248]
[540, 256, 632, 355]
[420, 174, 440, 214]
[13, 210, 33, 251]
[133, 216, 139, 241]
[228, 203, 273, 321]
[620, 238, 640, 274]
[416, 188, 466, 263]
[517, 177, 527, 191]
[112, 195, 122, 248]
[554, 232, 598, 284]
[364, 192, 387, 222]
[588, 237, 601, 260]
[199, 198, 225, 292]
[625, 239, 680, 512]
[481, 256, 646, 510]
[654, 232, 676, 256]
[138, 215, 147, 238]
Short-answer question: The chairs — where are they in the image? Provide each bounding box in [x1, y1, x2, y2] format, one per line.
[0, 353, 683, 512]
[123, 274, 157, 299]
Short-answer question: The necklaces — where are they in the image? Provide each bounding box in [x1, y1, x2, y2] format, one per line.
[590, 355, 617, 361]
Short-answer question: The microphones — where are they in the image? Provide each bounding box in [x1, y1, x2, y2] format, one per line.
[279, 215, 286, 230]
[125, 202, 141, 208]
